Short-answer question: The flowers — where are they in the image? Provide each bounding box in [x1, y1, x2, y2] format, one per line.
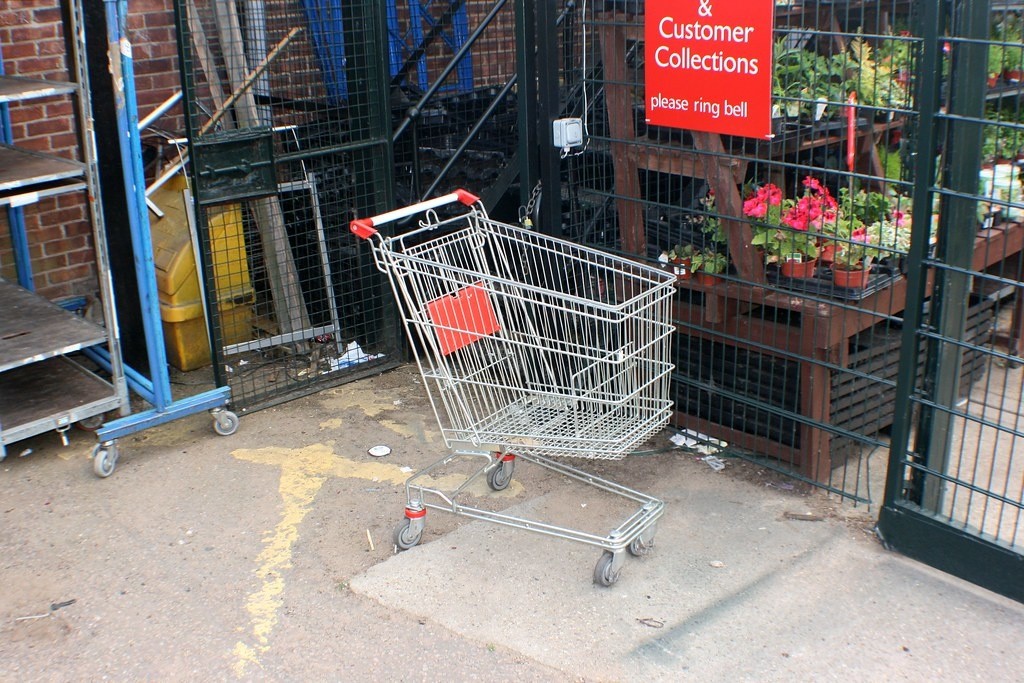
[688, 107, 1024, 268]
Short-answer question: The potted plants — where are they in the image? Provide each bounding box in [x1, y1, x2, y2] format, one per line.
[668, 247, 699, 280]
[690, 248, 728, 286]
[984, 32, 1024, 89]
[733, 37, 876, 160]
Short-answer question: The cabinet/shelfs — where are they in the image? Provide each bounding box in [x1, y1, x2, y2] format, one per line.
[0, 0, 133, 456]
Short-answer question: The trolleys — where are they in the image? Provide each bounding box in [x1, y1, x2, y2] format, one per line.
[348, 188, 678, 587]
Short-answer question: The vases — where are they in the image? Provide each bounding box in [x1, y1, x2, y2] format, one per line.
[985, 207, 1003, 227]
[830, 262, 872, 291]
[885, 243, 937, 271]
[779, 253, 817, 279]
